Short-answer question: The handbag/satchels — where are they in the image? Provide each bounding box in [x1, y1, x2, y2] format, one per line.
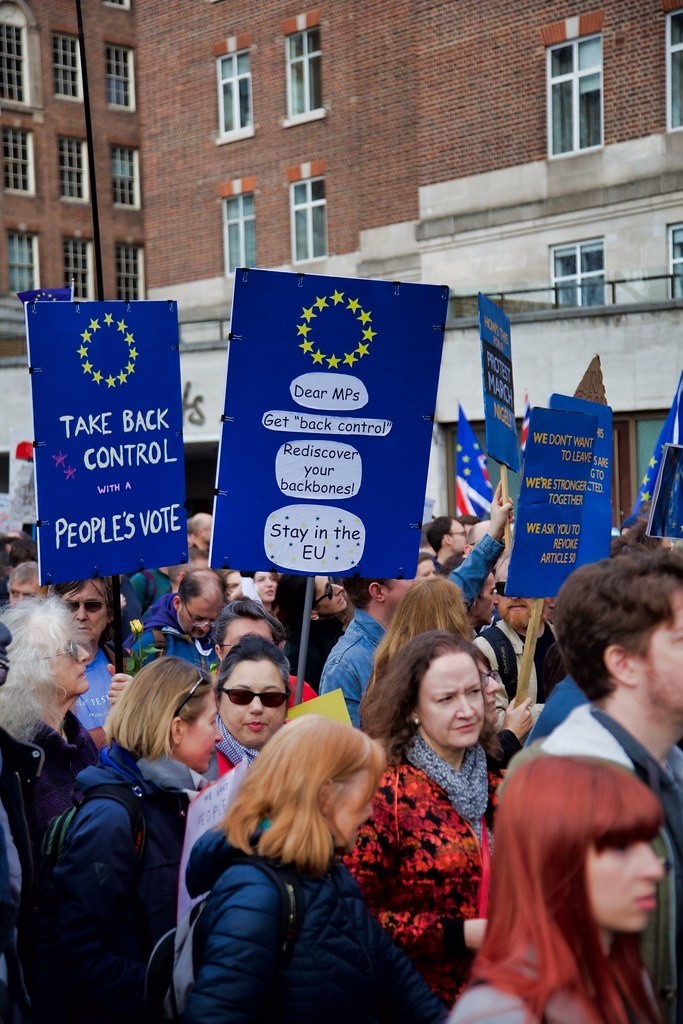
[156, 856, 305, 1023]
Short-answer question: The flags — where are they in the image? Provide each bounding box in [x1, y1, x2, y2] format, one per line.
[454, 411, 493, 519]
[620, 367, 683, 529]
[520, 396, 531, 465]
[17, 287, 71, 323]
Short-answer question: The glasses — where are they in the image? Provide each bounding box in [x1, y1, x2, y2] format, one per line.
[480, 670, 501, 687]
[173, 668, 211, 718]
[494, 581, 507, 596]
[220, 685, 289, 708]
[41, 639, 77, 660]
[182, 599, 217, 628]
[312, 576, 334, 610]
[65, 599, 106, 613]
[450, 530, 467, 538]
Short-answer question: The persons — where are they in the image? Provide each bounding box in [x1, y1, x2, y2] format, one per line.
[0, 501, 683, 1024]
